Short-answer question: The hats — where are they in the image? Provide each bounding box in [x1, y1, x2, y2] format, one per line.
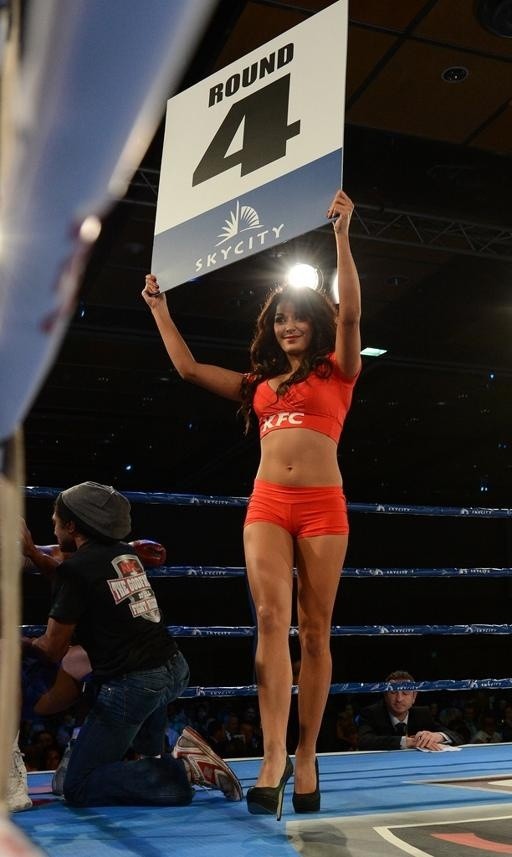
[61, 481, 132, 539]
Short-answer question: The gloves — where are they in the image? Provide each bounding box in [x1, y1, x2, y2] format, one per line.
[128, 540, 167, 566]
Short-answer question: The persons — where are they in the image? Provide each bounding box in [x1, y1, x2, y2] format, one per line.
[19, 481, 244, 808]
[17, 655, 512, 771]
[6, 537, 167, 813]
[140, 190, 363, 824]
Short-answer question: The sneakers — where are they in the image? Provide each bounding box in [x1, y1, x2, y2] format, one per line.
[173, 726, 243, 802]
[8, 749, 33, 812]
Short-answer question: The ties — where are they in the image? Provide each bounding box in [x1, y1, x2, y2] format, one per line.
[396, 722, 407, 736]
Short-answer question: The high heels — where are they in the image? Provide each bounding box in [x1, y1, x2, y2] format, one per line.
[246, 754, 293, 821]
[292, 757, 320, 813]
